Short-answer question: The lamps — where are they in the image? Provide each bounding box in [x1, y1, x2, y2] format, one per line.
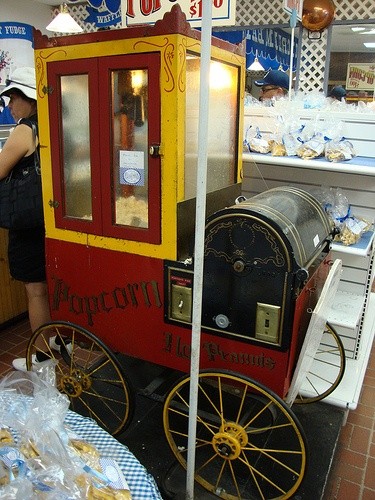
[246, 49, 266, 72]
[46, 1, 84, 34]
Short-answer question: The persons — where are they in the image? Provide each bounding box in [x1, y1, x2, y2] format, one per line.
[254, 69, 289, 103]
[331, 87, 346, 103]
[0, 67, 73, 376]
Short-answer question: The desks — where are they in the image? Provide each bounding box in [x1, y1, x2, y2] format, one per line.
[0, 392, 163, 500]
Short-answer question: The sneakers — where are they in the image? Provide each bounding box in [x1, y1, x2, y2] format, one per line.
[49, 335, 72, 354]
[11, 354, 55, 372]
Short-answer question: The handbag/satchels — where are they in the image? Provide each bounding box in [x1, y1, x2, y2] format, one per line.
[0, 119, 44, 229]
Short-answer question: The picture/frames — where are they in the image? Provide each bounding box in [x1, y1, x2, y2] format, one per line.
[345, 63, 375, 91]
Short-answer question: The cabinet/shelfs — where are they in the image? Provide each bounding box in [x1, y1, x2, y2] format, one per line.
[245, 102, 375, 426]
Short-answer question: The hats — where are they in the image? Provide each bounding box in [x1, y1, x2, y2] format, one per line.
[331, 87, 346, 98]
[253, 70, 289, 90]
[0, 67, 37, 100]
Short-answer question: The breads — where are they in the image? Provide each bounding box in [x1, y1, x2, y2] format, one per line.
[0, 427, 132, 500]
[269, 139, 355, 161]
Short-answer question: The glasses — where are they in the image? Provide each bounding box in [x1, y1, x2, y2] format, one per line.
[261, 87, 279, 94]
[5, 79, 36, 90]
